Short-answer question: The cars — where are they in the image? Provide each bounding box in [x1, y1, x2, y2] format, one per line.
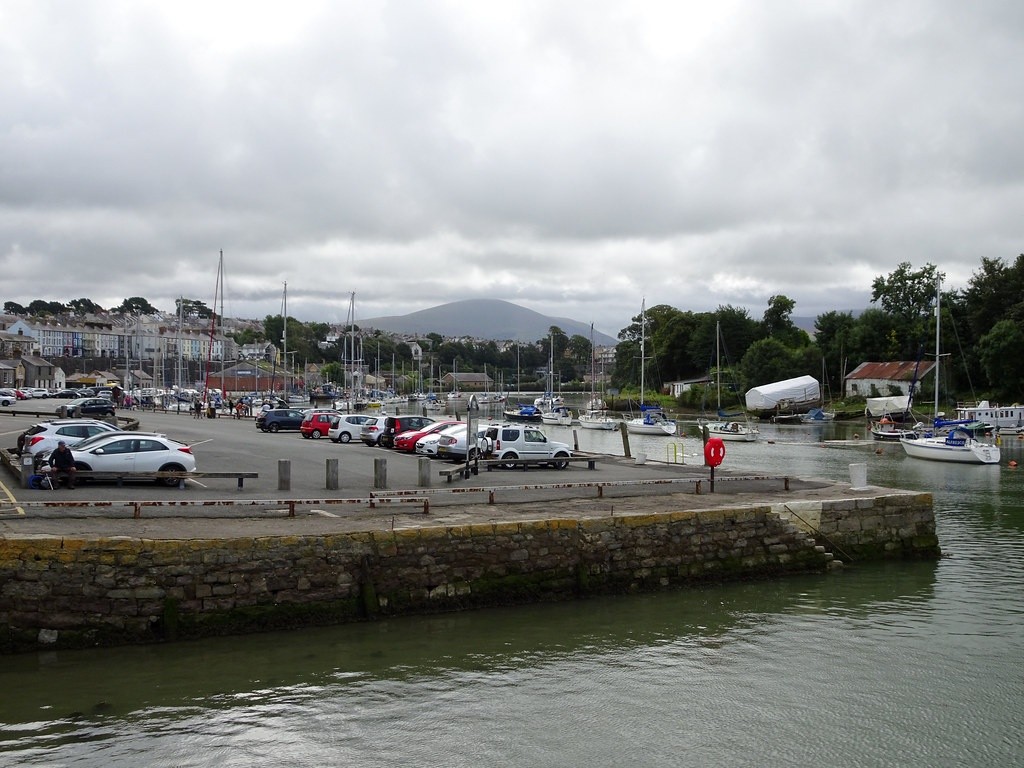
[438, 426, 491, 461]
[328, 414, 372, 443]
[300, 413, 340, 439]
[392, 420, 467, 455]
[0, 385, 197, 488]
[415, 424, 483, 459]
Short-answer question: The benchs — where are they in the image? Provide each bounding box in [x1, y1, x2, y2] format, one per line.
[439, 460, 478, 484]
[479, 456, 606, 471]
[38, 472, 259, 490]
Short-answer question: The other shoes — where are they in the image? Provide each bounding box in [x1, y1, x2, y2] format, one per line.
[67, 484, 76, 490]
[51, 483, 61, 490]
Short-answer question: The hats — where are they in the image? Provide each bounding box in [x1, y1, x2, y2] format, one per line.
[58, 440, 68, 446]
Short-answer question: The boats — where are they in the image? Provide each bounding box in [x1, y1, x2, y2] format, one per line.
[996, 425, 1024, 436]
[744, 345, 922, 423]
[898, 272, 1001, 465]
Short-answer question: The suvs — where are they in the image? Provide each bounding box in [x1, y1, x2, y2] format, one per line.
[256, 410, 306, 433]
[359, 417, 390, 447]
[480, 423, 571, 469]
[382, 415, 437, 449]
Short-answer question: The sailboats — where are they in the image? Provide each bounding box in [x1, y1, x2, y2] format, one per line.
[578, 322, 626, 430]
[621, 298, 677, 435]
[586, 349, 608, 411]
[869, 344, 924, 441]
[697, 321, 759, 442]
[115, 248, 574, 427]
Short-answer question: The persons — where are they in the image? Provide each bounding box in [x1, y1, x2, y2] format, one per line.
[194, 400, 274, 419]
[48, 441, 77, 490]
